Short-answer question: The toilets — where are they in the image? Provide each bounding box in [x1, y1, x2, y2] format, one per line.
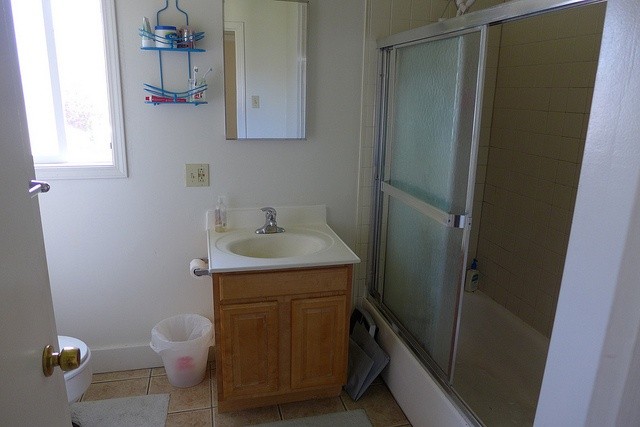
[57, 335, 92, 404]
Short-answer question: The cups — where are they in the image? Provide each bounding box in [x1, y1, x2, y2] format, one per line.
[188, 78, 206, 102]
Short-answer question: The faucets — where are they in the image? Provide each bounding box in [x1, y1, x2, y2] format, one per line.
[255, 207, 285, 234]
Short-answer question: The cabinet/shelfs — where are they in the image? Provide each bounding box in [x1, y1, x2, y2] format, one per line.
[211, 263, 355, 414]
[138, 0, 210, 107]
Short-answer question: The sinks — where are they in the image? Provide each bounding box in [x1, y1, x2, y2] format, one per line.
[227, 235, 326, 258]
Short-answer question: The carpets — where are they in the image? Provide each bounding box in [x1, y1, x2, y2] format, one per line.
[68, 392, 171, 427]
[249, 408, 374, 426]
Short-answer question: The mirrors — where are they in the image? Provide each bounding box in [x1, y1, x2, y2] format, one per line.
[222, 1, 307, 140]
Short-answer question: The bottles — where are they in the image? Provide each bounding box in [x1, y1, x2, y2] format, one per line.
[180, 26, 195, 48]
[155, 25, 178, 49]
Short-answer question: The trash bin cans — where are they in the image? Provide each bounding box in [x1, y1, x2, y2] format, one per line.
[151, 313, 213, 388]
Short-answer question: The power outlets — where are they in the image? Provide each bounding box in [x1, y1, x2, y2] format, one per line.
[186, 164, 211, 187]
[252, 96, 259, 108]
